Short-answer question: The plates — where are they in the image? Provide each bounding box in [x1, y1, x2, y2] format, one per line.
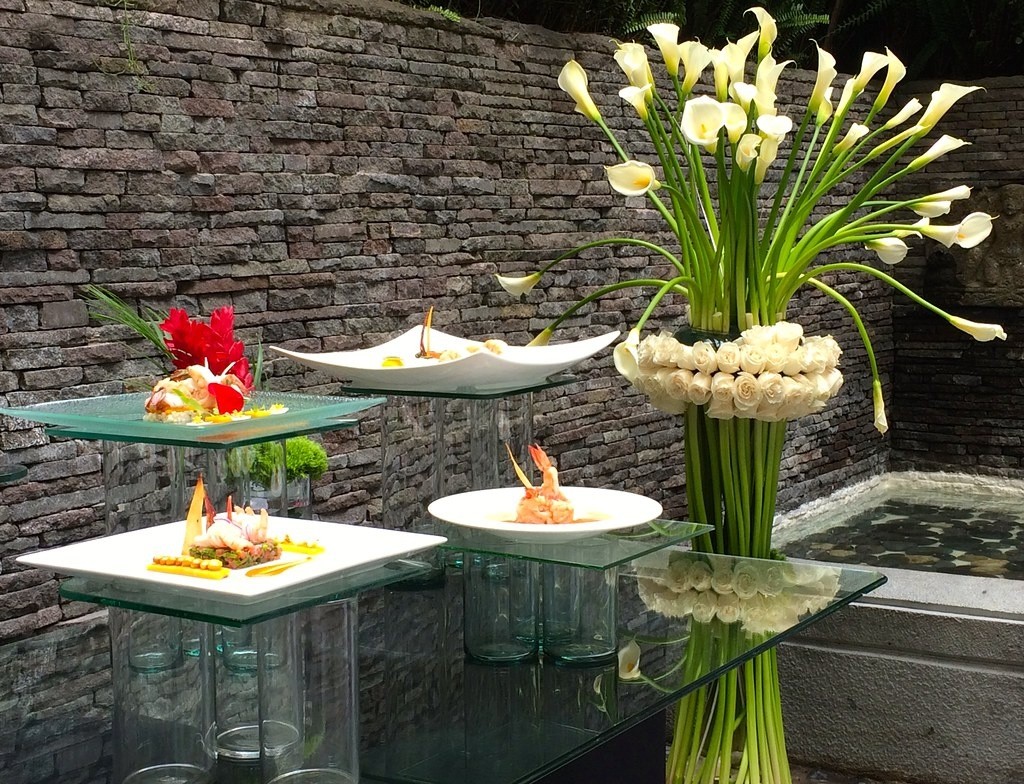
[428, 485, 663, 542]
[270, 325, 620, 387]
[17, 512, 448, 606]
[0, 391, 388, 437]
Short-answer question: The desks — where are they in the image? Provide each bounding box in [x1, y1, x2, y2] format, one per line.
[209, 541, 888, 784]
[0, 541, 887, 784]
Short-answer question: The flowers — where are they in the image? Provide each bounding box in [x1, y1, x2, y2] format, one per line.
[632, 543, 841, 783]
[494, 7, 1007, 560]
